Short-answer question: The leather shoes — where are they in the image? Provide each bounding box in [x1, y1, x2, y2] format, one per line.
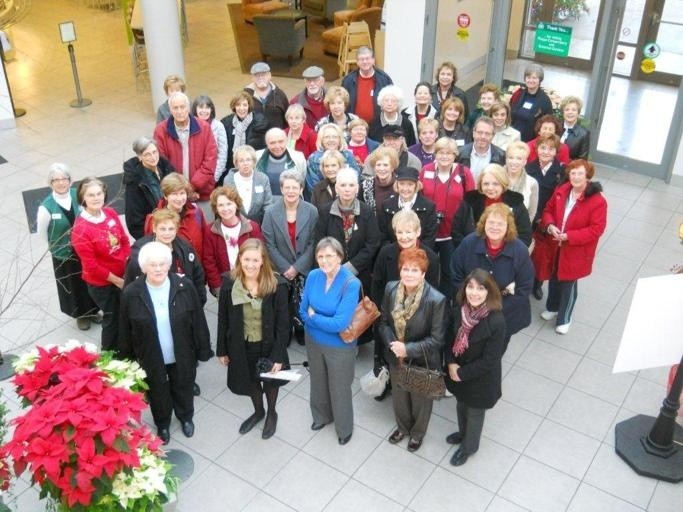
[449, 444, 478, 466]
[446, 432, 464, 445]
[311, 421, 323, 431]
[182, 419, 195, 438]
[91, 310, 105, 324]
[533, 281, 544, 301]
[337, 433, 351, 446]
[239, 410, 266, 434]
[389, 430, 404, 444]
[191, 382, 201, 397]
[78, 316, 90, 330]
[156, 426, 171, 446]
[261, 413, 278, 439]
[406, 437, 421, 452]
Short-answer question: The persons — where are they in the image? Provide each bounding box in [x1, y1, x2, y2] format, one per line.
[439, 269, 509, 466]
[372, 168, 435, 252]
[526, 135, 566, 300]
[313, 166, 377, 294]
[257, 127, 307, 199]
[425, 60, 467, 121]
[342, 117, 378, 164]
[366, 126, 419, 173]
[70, 176, 132, 352]
[142, 172, 204, 288]
[221, 145, 271, 223]
[468, 82, 500, 129]
[497, 141, 535, 220]
[451, 163, 532, 252]
[120, 241, 211, 444]
[362, 149, 400, 208]
[217, 239, 290, 438]
[262, 170, 317, 347]
[306, 123, 360, 190]
[403, 80, 437, 141]
[276, 105, 315, 155]
[533, 159, 607, 334]
[486, 102, 519, 153]
[37, 162, 106, 330]
[193, 97, 226, 187]
[220, 94, 266, 169]
[520, 115, 569, 162]
[416, 136, 472, 296]
[149, 93, 218, 204]
[373, 211, 439, 403]
[432, 97, 465, 137]
[315, 86, 357, 136]
[240, 62, 289, 127]
[297, 237, 360, 445]
[122, 137, 177, 239]
[341, 47, 395, 123]
[314, 151, 362, 207]
[507, 62, 553, 141]
[408, 117, 437, 162]
[453, 116, 506, 188]
[379, 251, 447, 452]
[451, 202, 532, 359]
[154, 77, 192, 125]
[555, 97, 591, 159]
[365, 83, 415, 147]
[121, 208, 207, 405]
[285, 66, 334, 121]
[202, 188, 266, 298]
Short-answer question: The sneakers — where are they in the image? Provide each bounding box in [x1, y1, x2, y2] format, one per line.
[541, 309, 559, 322]
[555, 318, 574, 335]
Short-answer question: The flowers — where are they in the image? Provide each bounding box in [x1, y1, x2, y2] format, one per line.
[0, 342, 181, 512]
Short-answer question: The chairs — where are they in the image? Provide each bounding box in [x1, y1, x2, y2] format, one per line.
[241, 0, 290, 23]
[321, 7, 381, 56]
[252, 15, 308, 66]
[334, 0, 385, 26]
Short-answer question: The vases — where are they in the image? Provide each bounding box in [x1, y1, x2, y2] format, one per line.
[162, 492, 177, 512]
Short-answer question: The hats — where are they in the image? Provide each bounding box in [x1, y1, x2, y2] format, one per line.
[374, 124, 405, 138]
[303, 65, 324, 79]
[395, 167, 421, 184]
[250, 62, 271, 75]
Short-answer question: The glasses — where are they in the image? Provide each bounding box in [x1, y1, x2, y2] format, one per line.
[52, 176, 69, 182]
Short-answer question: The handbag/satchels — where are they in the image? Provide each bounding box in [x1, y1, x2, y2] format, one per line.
[395, 343, 445, 399]
[341, 275, 380, 342]
